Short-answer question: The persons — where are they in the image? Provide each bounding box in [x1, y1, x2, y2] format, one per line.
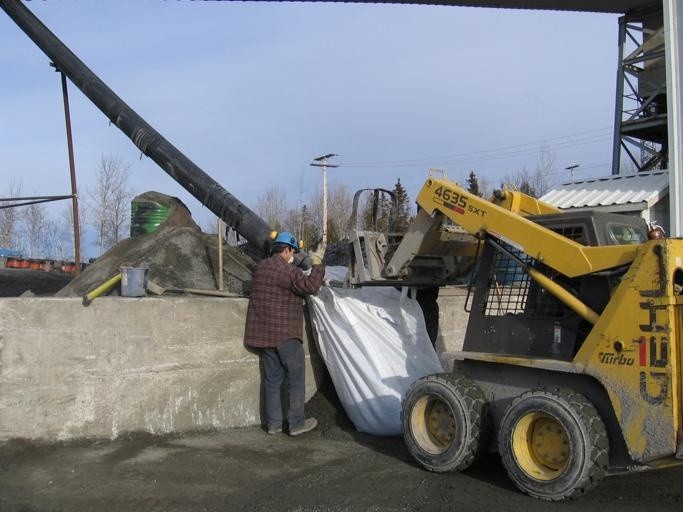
[244, 232, 325, 436]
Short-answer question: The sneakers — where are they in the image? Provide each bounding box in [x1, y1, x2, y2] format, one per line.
[268, 416, 318, 435]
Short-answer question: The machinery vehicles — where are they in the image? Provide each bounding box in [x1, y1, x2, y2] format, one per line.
[345, 168, 683, 503]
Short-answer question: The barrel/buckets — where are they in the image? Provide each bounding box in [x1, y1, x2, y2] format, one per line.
[130, 201, 175, 237]
[119, 263, 147, 297]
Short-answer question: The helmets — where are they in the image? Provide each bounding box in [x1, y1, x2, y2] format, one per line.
[273, 232, 301, 252]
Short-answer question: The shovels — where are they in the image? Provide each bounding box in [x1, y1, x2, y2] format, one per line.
[145, 280, 242, 297]
